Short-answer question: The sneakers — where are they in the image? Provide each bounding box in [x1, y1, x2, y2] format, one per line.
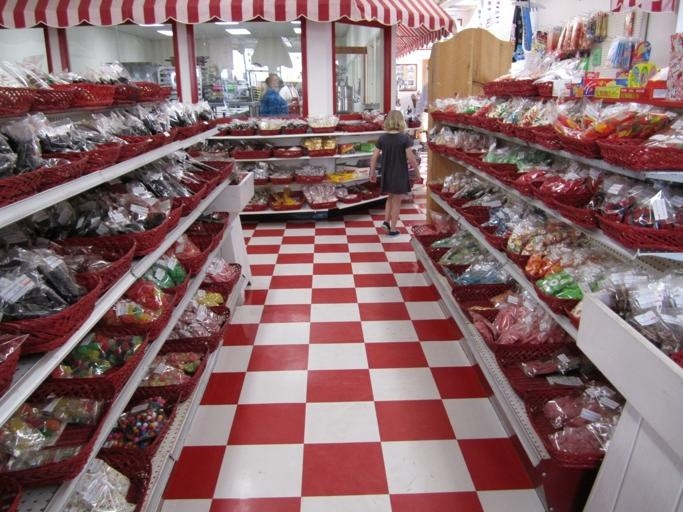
[389, 231, 400, 235]
[381, 221, 390, 230]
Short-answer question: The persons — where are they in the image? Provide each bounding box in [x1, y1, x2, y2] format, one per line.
[287, 81, 299, 99]
[396, 90, 421, 120]
[277, 79, 292, 103]
[369, 110, 420, 236]
[259, 75, 288, 115]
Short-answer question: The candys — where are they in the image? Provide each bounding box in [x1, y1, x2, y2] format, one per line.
[0, 302, 198, 449]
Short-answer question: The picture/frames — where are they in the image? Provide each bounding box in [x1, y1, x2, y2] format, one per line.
[396, 63, 419, 91]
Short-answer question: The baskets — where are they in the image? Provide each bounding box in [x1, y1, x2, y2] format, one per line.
[234, 114, 423, 210]
[0, 80, 240, 511]
[412, 77, 682, 470]
